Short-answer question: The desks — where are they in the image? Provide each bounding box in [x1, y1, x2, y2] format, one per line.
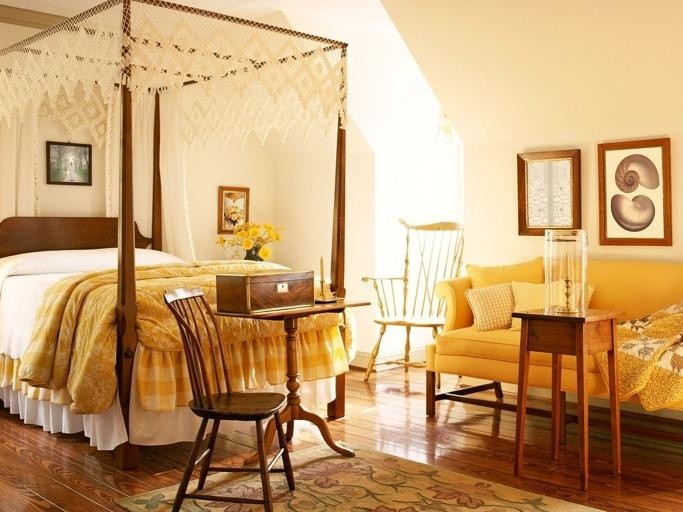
[203, 301, 372, 468]
[511, 305, 621, 492]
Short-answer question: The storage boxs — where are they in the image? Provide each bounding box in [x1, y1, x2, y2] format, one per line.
[216, 271, 314, 315]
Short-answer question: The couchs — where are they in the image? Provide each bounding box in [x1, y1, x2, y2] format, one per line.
[424, 255, 682, 423]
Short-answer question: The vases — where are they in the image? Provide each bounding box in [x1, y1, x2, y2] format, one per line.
[242, 246, 265, 262]
[544, 229, 587, 316]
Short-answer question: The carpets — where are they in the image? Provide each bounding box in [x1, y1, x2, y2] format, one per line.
[115, 440, 615, 511]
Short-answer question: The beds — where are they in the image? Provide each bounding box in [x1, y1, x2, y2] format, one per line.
[0, 0, 349, 451]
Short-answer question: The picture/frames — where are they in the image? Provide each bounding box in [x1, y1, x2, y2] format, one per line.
[45, 141, 90, 187]
[217, 187, 250, 234]
[597, 138, 672, 247]
[516, 148, 581, 236]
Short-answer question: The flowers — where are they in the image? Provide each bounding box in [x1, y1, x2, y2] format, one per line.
[213, 222, 282, 259]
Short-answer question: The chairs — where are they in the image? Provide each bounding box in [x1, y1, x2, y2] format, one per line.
[361, 219, 468, 388]
[162, 286, 294, 510]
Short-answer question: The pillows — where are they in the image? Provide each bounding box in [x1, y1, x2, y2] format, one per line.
[551, 278, 596, 309]
[511, 280, 561, 330]
[463, 283, 513, 332]
